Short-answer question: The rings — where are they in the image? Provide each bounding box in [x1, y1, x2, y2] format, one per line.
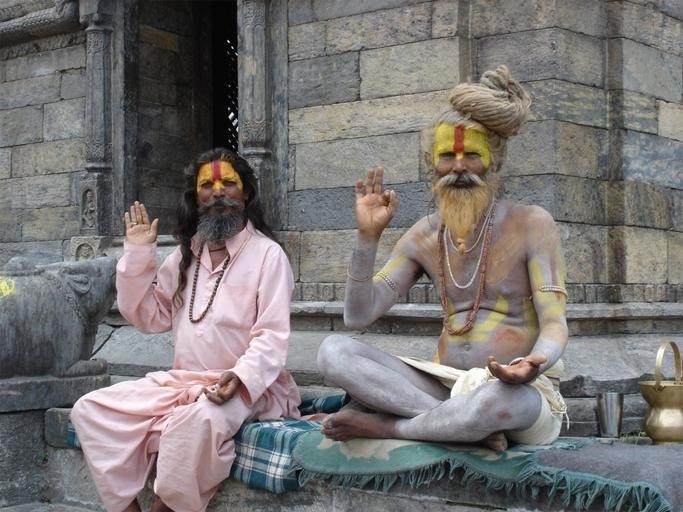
[130, 221, 137, 225]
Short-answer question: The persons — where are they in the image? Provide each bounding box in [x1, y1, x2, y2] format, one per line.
[69, 148, 301, 512]
[313, 63, 567, 451]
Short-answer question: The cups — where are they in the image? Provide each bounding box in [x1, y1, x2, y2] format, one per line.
[596, 393, 624, 438]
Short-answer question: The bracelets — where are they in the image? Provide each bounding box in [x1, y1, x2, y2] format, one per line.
[345, 264, 371, 282]
[509, 356, 524, 365]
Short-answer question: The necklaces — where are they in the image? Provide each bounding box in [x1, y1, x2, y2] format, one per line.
[208, 246, 225, 253]
[188, 237, 229, 323]
[435, 203, 495, 334]
[447, 211, 488, 253]
[442, 202, 491, 289]
